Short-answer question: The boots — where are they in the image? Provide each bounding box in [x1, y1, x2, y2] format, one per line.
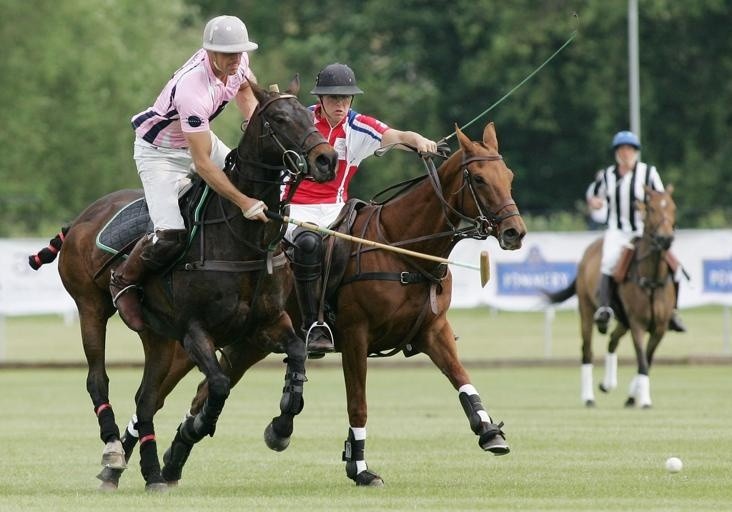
[109, 229, 187, 332]
[292, 222, 335, 353]
[669, 282, 685, 333]
[596, 274, 618, 334]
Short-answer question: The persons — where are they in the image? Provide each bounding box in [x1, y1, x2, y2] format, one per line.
[108, 15, 272, 332]
[585, 130, 688, 333]
[280, 63, 438, 359]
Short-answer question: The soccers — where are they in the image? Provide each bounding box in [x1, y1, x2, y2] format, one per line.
[666, 458, 681, 474]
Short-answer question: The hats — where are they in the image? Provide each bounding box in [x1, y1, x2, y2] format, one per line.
[310, 64, 364, 95]
[613, 130, 640, 150]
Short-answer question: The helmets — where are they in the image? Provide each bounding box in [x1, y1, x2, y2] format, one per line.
[203, 15, 259, 53]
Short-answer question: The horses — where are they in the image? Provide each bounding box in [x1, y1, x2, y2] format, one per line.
[28, 74, 339, 493]
[539, 181, 675, 408]
[98, 121, 526, 486]
